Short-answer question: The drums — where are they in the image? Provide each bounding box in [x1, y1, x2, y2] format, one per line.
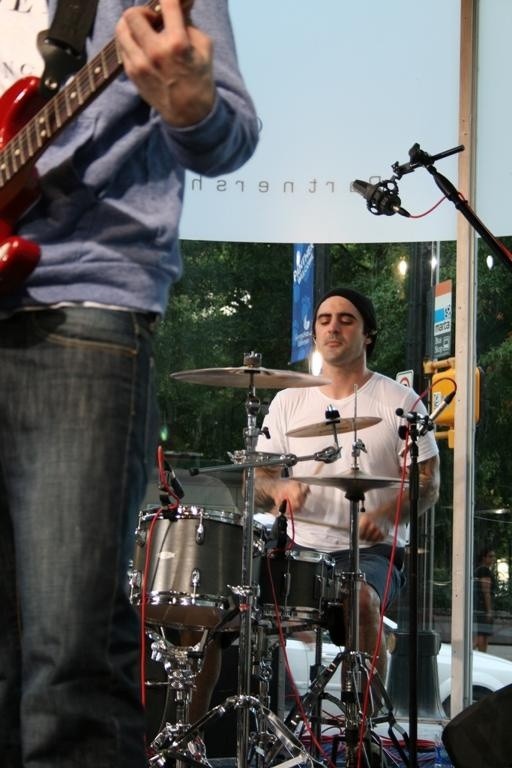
[129, 504, 266, 634]
[255, 549, 336, 630]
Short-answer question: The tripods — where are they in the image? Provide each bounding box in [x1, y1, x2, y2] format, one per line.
[150, 398, 307, 768]
[294, 492, 410, 768]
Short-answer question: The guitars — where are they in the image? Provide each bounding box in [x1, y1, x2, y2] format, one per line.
[1, 0, 194, 296]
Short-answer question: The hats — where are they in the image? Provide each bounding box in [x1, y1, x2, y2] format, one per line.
[313, 287, 378, 334]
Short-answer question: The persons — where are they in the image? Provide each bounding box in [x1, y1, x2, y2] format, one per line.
[0, 0, 260, 768]
[187, 288, 442, 762]
[473, 546, 497, 653]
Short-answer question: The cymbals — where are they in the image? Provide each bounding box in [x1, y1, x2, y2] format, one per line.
[285, 416, 381, 438]
[171, 367, 330, 390]
[289, 469, 409, 489]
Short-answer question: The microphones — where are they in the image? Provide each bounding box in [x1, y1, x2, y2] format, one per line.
[396, 390, 458, 458]
[351, 178, 411, 220]
[156, 457, 185, 500]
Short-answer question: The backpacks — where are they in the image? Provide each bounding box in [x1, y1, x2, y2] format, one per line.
[474, 579, 481, 610]
